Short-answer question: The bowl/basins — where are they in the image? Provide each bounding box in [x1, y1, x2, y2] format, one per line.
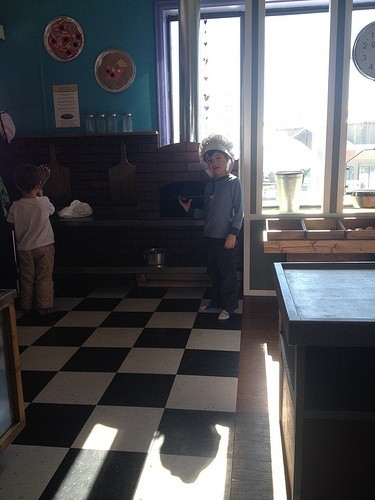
[346, 191, 375, 208]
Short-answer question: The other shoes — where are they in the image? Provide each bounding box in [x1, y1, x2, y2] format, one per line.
[200, 298, 219, 309]
[218, 308, 234, 320]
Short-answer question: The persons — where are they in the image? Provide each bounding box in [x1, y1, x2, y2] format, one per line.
[0, 110, 18, 296]
[179, 137, 244, 320]
[6, 163, 57, 317]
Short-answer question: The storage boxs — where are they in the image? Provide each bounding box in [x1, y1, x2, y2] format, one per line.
[265, 218, 305, 240]
[303, 218, 345, 240]
[338, 217, 375, 240]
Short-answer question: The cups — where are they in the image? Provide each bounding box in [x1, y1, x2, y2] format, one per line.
[275, 171, 303, 212]
[122, 113, 132, 132]
[86, 114, 95, 132]
[97, 113, 106, 132]
[108, 113, 117, 132]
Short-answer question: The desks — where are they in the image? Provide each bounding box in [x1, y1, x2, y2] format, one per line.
[274, 262, 375, 500]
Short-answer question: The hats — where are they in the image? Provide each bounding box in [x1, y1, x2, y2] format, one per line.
[199, 134, 235, 178]
